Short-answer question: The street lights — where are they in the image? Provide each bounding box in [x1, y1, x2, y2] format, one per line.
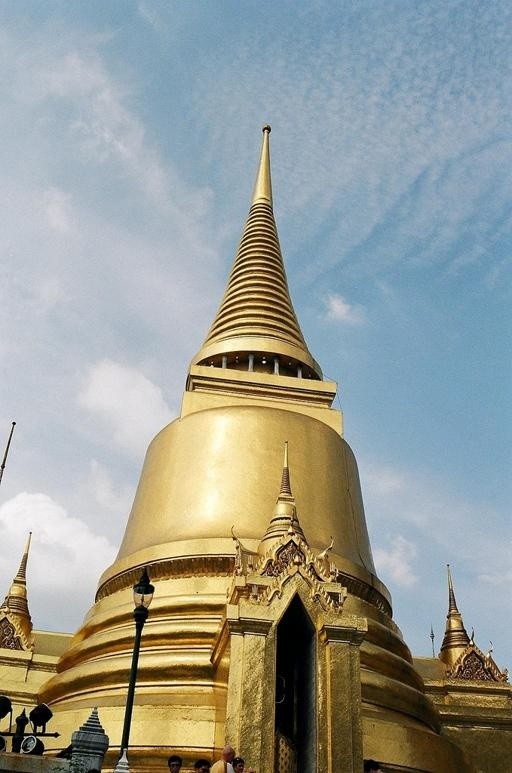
[117, 564, 156, 766]
